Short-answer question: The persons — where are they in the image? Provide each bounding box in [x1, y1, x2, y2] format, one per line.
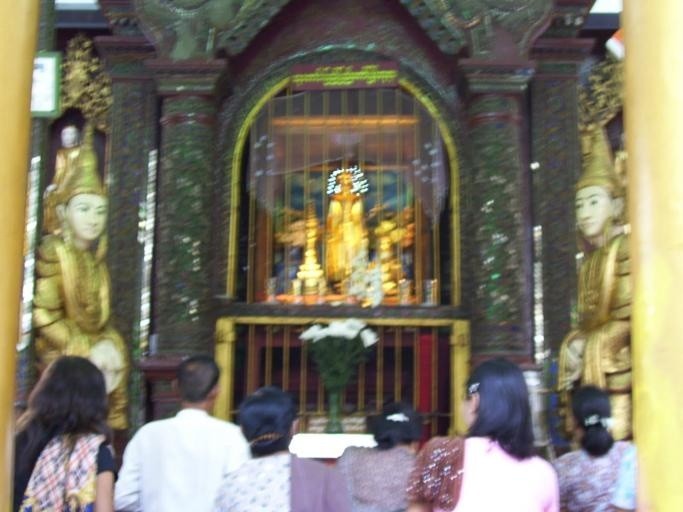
[41, 122, 80, 233]
[35, 125, 130, 430]
[550, 384, 635, 511]
[403, 357, 559, 512]
[10, 354, 122, 510]
[114, 354, 254, 511]
[209, 382, 350, 511]
[332, 400, 423, 512]
[549, 126, 634, 448]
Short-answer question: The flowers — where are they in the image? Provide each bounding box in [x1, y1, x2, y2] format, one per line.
[298, 316, 381, 428]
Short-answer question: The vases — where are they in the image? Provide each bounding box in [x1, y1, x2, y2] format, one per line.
[323, 385, 343, 433]
